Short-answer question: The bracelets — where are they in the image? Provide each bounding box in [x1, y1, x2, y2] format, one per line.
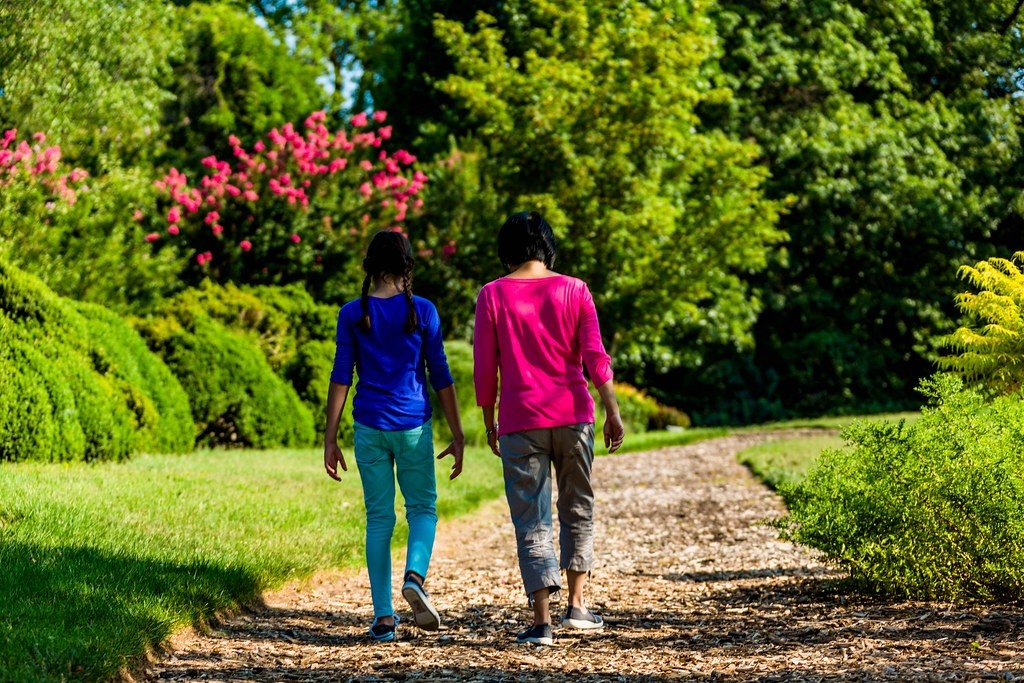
[484, 424, 497, 435]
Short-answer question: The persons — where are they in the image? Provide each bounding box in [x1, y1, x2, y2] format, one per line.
[320, 229, 463, 642]
[471, 210, 626, 645]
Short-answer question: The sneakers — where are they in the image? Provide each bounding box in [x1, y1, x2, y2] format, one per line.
[402, 575, 440, 631]
[369, 613, 400, 641]
[517, 622, 552, 646]
[563, 605, 603, 628]
